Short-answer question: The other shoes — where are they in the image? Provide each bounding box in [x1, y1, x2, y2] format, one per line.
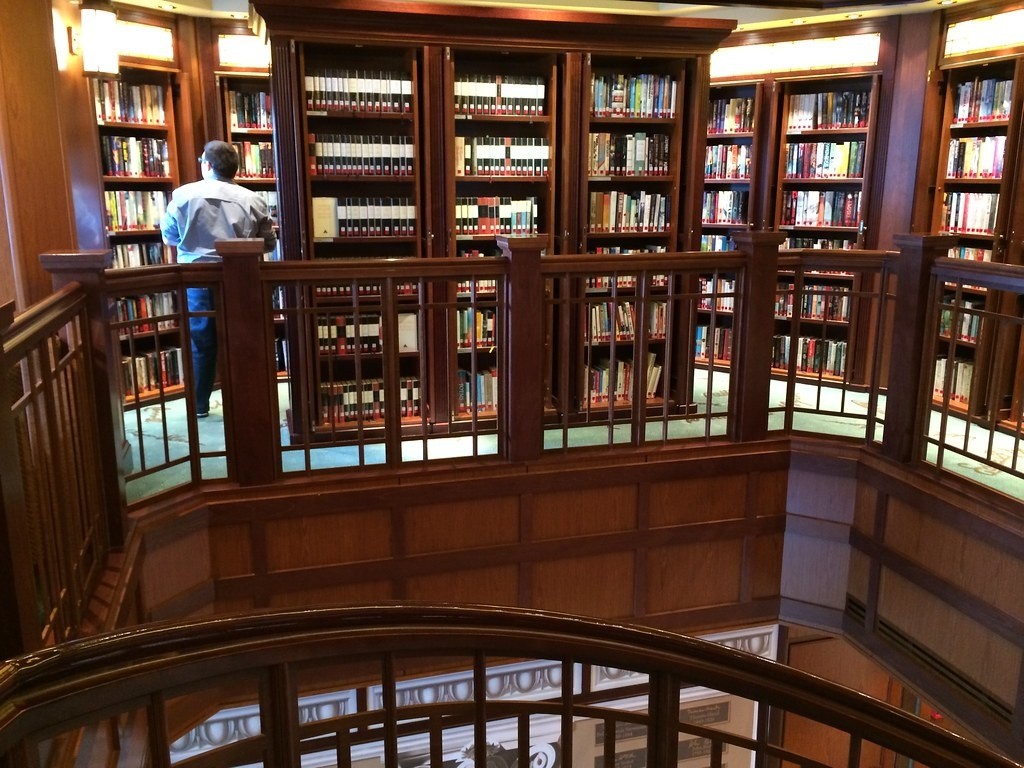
[196, 410, 209, 417]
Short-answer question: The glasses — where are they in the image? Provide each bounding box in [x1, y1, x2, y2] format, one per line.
[198, 157, 213, 168]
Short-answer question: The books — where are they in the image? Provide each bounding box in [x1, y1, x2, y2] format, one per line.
[583, 62, 1014, 407]
[76, 64, 278, 395]
[302, 56, 553, 427]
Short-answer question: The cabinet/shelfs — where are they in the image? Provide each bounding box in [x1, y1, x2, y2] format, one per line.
[929, 49, 1024, 440]
[696, 66, 881, 392]
[0, 1, 289, 414]
[247, 0, 738, 451]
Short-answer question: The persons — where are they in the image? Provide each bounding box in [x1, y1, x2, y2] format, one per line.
[160, 141, 277, 417]
